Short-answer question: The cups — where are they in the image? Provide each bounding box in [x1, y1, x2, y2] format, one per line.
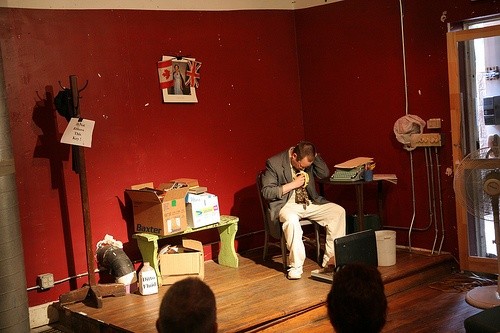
[359, 170, 373, 181]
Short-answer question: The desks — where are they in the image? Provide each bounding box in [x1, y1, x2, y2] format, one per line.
[317, 175, 376, 231]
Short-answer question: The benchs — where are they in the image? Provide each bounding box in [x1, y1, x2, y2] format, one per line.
[132, 214, 240, 288]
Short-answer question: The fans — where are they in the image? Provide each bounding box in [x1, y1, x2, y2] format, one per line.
[453, 145, 500, 309]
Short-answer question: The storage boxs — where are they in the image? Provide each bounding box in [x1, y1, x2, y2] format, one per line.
[183, 194, 220, 229]
[157, 238, 205, 285]
[125, 181, 189, 237]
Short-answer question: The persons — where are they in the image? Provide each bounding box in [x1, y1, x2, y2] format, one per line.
[155, 277, 218, 333]
[261, 143, 346, 279]
[327, 267, 387, 333]
[173, 64, 186, 95]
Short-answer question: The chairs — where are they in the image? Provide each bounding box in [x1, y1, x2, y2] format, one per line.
[256, 170, 321, 274]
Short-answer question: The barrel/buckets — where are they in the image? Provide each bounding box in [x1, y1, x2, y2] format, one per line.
[349, 215, 380, 235]
[374, 230, 396, 267]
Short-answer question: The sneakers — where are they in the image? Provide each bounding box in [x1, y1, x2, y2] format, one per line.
[287, 263, 303, 280]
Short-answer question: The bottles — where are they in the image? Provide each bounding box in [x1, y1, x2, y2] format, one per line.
[138, 262, 158, 296]
[167, 218, 172, 232]
[176, 216, 180, 227]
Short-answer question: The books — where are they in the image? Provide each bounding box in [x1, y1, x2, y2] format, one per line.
[334, 157, 375, 169]
[373, 174, 398, 184]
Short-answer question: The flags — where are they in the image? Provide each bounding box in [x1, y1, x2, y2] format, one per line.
[158, 61, 174, 89]
[185, 61, 201, 89]
